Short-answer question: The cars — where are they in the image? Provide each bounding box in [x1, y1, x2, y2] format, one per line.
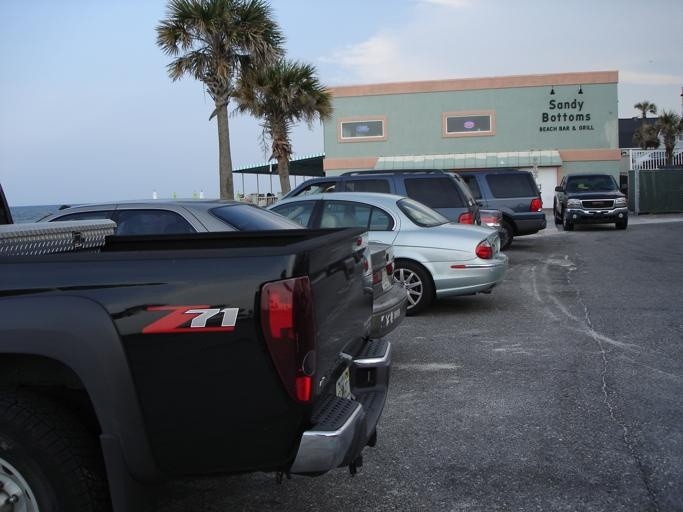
[33, 200, 304, 237]
[265, 192, 508, 310]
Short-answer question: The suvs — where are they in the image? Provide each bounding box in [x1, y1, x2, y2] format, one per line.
[554, 172, 639, 231]
[271, 172, 481, 220]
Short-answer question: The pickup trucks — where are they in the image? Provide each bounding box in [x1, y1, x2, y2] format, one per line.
[1, 178, 410, 504]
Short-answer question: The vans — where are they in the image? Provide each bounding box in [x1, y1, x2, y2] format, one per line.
[458, 169, 548, 247]
